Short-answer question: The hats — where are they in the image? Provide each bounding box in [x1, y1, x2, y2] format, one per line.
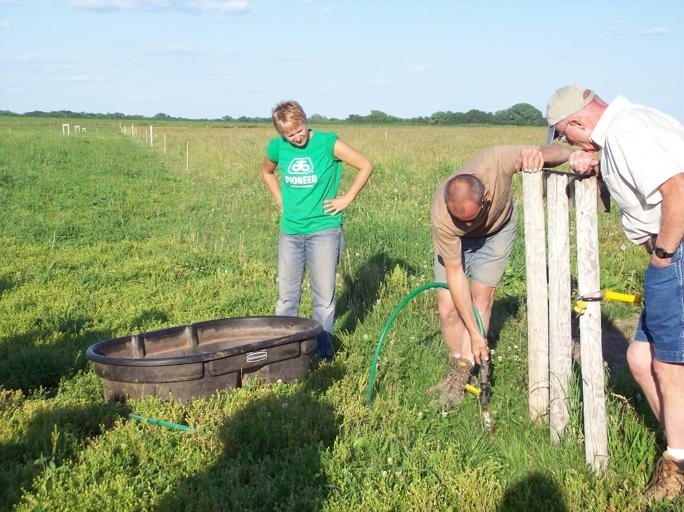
[545, 86, 595, 147]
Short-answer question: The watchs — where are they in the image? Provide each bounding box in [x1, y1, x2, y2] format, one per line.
[653, 245, 674, 259]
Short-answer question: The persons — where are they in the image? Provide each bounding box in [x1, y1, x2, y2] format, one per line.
[421, 142, 571, 410]
[260, 100, 374, 363]
[545, 84, 684, 512]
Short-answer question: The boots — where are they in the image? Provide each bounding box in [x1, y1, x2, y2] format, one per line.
[426, 356, 477, 395]
[440, 358, 473, 404]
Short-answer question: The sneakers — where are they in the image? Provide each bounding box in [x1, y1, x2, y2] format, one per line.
[642, 450, 684, 501]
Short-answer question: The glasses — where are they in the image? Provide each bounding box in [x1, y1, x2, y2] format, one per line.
[558, 122, 569, 143]
